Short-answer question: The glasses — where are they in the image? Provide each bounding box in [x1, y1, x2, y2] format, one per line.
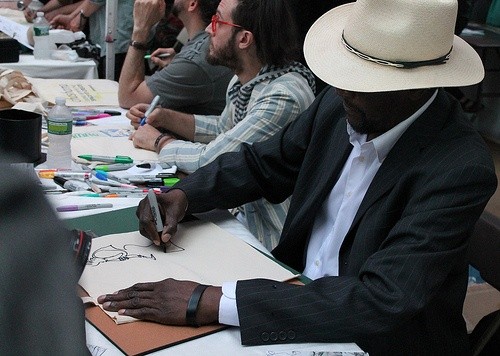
[211, 15, 245, 33]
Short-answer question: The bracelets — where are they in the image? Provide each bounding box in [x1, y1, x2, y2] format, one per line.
[185, 283, 212, 327]
[80, 9, 90, 19]
[155, 132, 174, 154]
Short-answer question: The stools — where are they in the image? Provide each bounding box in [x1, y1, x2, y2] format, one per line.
[463, 37, 500, 122]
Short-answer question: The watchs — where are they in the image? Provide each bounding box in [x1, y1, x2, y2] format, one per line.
[129, 40, 149, 50]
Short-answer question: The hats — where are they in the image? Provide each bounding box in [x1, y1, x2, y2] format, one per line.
[302, 0, 486, 93]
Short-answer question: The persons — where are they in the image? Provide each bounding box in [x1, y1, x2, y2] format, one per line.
[124, 0, 318, 254]
[15, 0, 187, 82]
[98, 0, 500, 356]
[118, 0, 235, 116]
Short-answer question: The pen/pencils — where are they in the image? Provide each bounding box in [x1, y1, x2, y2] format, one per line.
[24, 5, 36, 23]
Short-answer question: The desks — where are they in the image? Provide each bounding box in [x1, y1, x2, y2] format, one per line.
[0, 0, 99, 80]
[0, 79, 371, 356]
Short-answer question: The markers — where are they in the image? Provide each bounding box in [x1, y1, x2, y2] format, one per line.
[139, 94, 161, 126]
[69, 107, 121, 126]
[92, 164, 134, 172]
[52, 167, 171, 197]
[78, 155, 133, 164]
[144, 53, 171, 58]
[148, 188, 167, 253]
[55, 203, 113, 212]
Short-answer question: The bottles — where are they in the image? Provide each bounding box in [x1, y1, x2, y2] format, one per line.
[46, 97, 73, 172]
[33, 11, 50, 63]
